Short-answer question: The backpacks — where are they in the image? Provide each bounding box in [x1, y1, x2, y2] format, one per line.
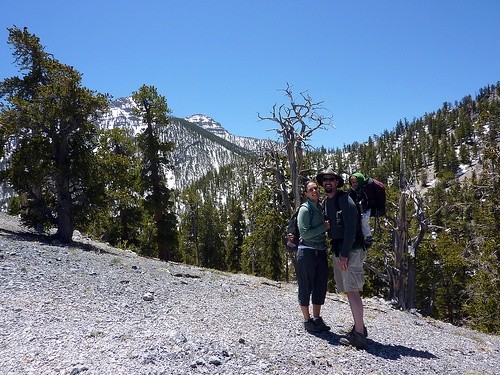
[359, 176, 386, 217]
[284, 204, 314, 252]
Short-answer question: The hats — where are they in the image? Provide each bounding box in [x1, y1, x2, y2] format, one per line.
[316, 167, 344, 188]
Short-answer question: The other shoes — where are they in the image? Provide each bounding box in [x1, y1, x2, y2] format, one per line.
[304, 319, 322, 333]
[336, 325, 368, 348]
[315, 317, 331, 331]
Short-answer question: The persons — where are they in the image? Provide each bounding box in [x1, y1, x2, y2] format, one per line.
[348, 172, 373, 250]
[316, 168, 368, 347]
[295, 180, 331, 333]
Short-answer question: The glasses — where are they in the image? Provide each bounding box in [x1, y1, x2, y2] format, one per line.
[323, 177, 336, 181]
